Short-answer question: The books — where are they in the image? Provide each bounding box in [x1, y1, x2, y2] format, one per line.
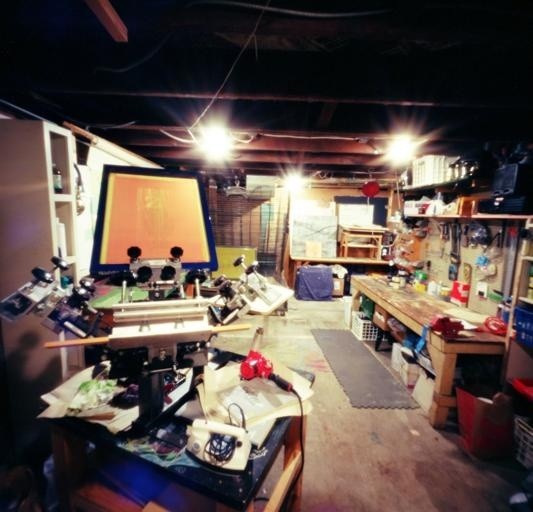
[216, 377, 314, 426]
[244, 419, 276, 448]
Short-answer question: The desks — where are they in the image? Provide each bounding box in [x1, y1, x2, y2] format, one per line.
[339, 224, 387, 260]
[36, 347, 316, 512]
[350, 274, 505, 429]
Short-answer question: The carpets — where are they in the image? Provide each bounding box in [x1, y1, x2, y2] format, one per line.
[311, 328, 420, 410]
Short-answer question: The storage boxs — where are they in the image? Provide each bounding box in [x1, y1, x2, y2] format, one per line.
[332, 278, 344, 296]
[514, 417, 533, 470]
[513, 305, 533, 348]
[350, 311, 379, 341]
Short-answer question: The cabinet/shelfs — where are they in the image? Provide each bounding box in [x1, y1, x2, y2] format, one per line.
[500, 219, 533, 394]
[1, 120, 79, 387]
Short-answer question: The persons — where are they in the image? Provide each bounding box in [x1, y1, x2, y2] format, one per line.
[151, 347, 171, 369]
